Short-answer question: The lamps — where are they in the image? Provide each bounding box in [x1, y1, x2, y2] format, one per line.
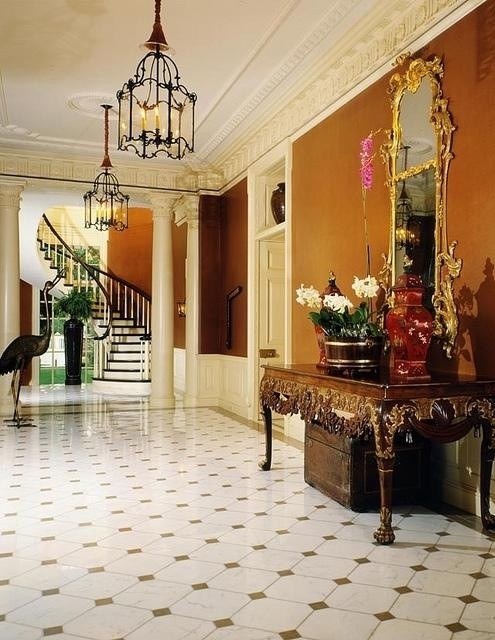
[82, 105, 131, 232]
[116, 0, 198, 160]
[395, 146, 421, 249]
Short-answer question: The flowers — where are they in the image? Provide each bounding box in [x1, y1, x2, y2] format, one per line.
[295, 275, 385, 336]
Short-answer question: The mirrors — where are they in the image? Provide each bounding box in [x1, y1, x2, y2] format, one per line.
[378, 52, 462, 359]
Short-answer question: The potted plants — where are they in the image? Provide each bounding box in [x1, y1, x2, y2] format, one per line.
[53, 291, 93, 385]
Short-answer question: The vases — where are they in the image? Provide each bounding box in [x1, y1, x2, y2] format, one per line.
[313, 269, 348, 369]
[323, 342, 377, 374]
[384, 274, 433, 386]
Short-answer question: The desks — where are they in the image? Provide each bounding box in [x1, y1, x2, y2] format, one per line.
[256, 364, 495, 546]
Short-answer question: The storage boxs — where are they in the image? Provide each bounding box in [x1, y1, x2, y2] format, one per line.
[303, 421, 444, 513]
